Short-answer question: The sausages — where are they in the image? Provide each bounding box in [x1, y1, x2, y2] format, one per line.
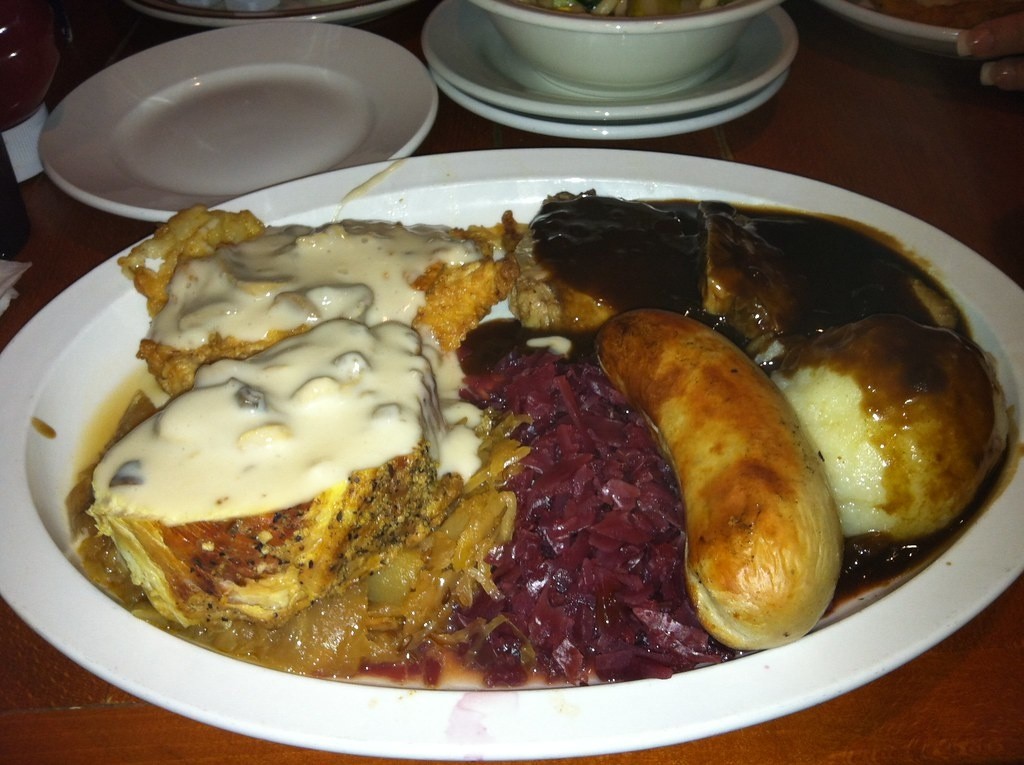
[593, 306, 841, 653]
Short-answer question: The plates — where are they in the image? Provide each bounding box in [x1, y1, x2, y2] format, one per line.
[421, 1, 798, 121]
[429, 66, 791, 142]
[818, 1, 969, 43]
[0, 148, 1023, 763]
[35, 22, 438, 220]
[129, 1, 412, 26]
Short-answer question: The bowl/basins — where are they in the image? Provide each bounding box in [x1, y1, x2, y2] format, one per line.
[468, 0, 787, 96]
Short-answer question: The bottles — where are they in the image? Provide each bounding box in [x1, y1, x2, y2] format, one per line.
[1, 0, 58, 183]
[0, 135, 31, 261]
